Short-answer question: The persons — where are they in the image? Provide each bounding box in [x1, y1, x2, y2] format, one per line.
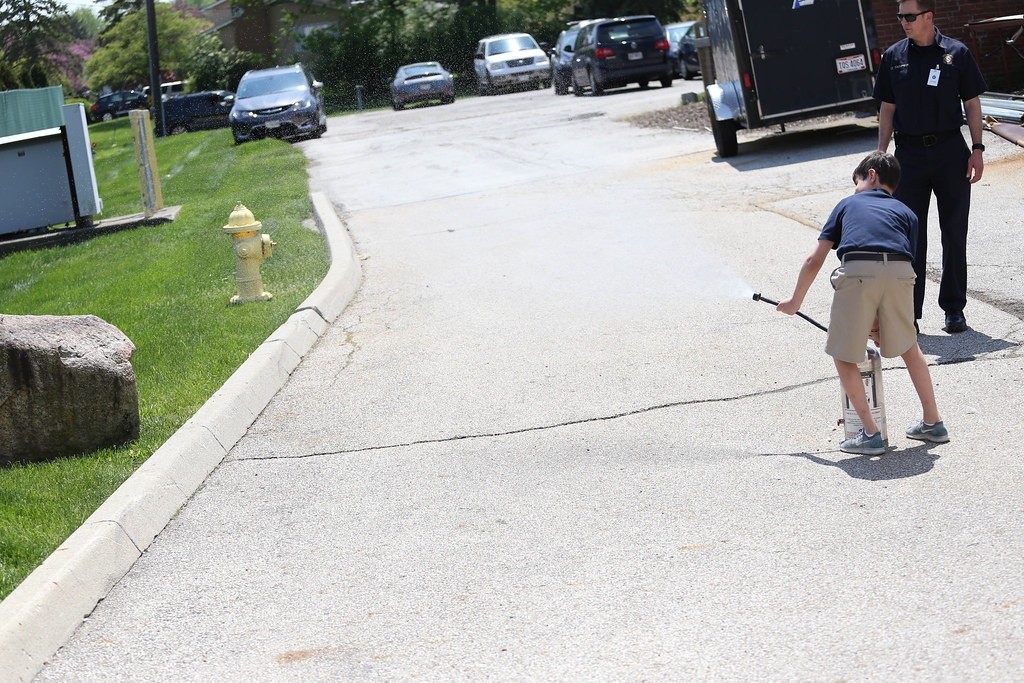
[776, 151, 948, 455]
[872, 0, 987, 335]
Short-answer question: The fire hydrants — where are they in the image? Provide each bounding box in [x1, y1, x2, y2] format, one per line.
[222, 200, 278, 305]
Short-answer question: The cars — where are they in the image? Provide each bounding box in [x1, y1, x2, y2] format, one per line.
[142, 81, 186, 104]
[547, 18, 600, 96]
[662, 21, 705, 81]
[388, 62, 455, 112]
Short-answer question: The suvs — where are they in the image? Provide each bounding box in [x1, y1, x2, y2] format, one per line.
[89, 90, 150, 122]
[154, 89, 236, 139]
[563, 14, 673, 97]
[223, 62, 327, 146]
[474, 33, 552, 95]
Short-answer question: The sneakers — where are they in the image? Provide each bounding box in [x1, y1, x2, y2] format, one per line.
[905, 420, 949, 442]
[840, 428, 885, 455]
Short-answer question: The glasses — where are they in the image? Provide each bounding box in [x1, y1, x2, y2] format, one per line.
[896, 10, 930, 22]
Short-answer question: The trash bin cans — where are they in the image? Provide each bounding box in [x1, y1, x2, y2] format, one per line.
[0, 102, 104, 240]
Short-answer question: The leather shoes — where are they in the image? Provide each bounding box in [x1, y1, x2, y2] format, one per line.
[945, 311, 967, 332]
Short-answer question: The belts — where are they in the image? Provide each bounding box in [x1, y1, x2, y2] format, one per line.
[893, 128, 960, 147]
[844, 253, 912, 262]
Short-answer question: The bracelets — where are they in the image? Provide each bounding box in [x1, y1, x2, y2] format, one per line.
[972, 143, 985, 152]
